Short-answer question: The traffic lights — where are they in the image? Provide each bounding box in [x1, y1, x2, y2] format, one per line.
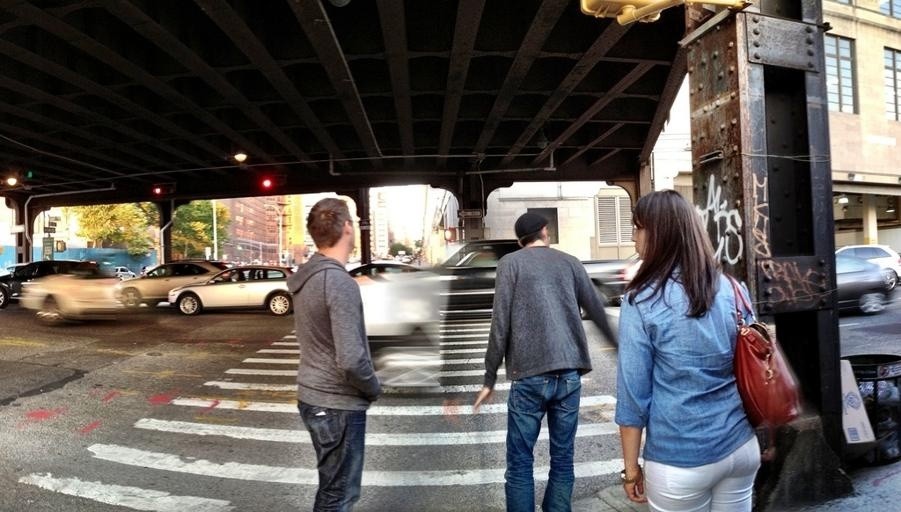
[263, 180, 271, 187]
[153, 183, 174, 194]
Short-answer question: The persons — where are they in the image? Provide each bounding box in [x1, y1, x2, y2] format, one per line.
[285, 197, 383, 510]
[612, 189, 765, 510]
[139, 266, 146, 277]
[472, 213, 621, 511]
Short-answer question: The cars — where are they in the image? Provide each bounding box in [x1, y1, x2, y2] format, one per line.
[833, 243, 901, 315]
[1, 261, 101, 308]
[115, 267, 136, 280]
[116, 259, 231, 312]
[19, 271, 123, 322]
[169, 266, 297, 315]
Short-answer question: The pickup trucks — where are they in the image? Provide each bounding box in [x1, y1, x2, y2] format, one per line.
[433, 241, 638, 319]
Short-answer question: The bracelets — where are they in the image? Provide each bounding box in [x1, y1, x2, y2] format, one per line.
[620, 464, 642, 485]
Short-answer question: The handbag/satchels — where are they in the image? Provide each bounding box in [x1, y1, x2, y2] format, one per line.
[733, 322, 802, 431]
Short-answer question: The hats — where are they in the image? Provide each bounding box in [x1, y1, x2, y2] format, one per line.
[515, 212, 549, 238]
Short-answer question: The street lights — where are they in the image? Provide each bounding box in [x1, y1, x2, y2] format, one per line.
[264, 204, 287, 262]
